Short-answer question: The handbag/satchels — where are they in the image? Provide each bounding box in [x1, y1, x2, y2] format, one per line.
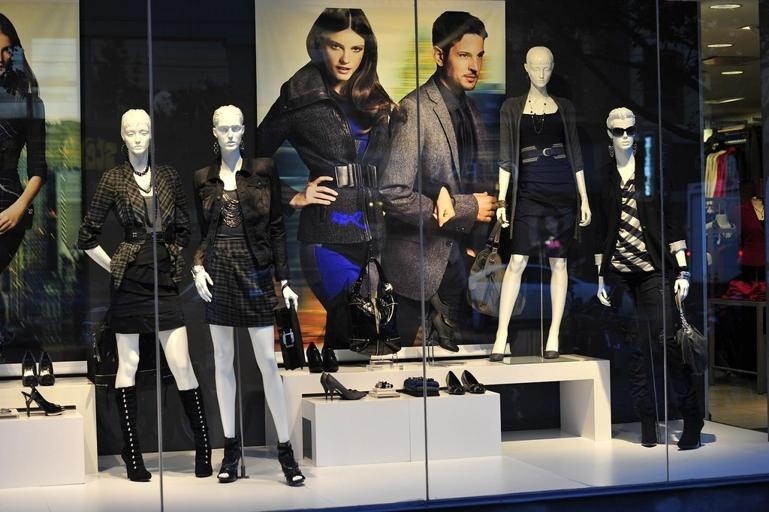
[274, 298, 305, 370]
[347, 257, 401, 357]
[674, 290, 708, 387]
[465, 217, 528, 317]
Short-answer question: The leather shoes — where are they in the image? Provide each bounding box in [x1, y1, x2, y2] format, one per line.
[306, 342, 324, 373]
[321, 342, 338, 372]
[543, 350, 559, 359]
[22, 349, 38, 386]
[461, 370, 486, 394]
[446, 371, 465, 395]
[491, 351, 504, 362]
[38, 351, 55, 386]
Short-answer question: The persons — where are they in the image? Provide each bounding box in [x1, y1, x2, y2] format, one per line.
[380, 11, 501, 349]
[0, 14, 45, 270]
[592, 108, 711, 448]
[76, 107, 212, 483]
[492, 43, 591, 362]
[188, 101, 306, 487]
[257, 8, 454, 354]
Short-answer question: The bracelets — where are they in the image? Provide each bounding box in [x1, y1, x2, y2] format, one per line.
[675, 266, 689, 279]
[496, 200, 506, 208]
[191, 266, 204, 278]
[282, 281, 291, 293]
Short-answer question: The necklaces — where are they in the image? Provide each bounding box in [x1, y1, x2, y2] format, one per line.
[527, 92, 550, 136]
[137, 181, 154, 194]
[126, 157, 152, 175]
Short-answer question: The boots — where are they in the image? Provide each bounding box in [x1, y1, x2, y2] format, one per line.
[277, 440, 305, 486]
[177, 385, 213, 477]
[114, 385, 151, 480]
[217, 435, 241, 483]
[641, 409, 657, 447]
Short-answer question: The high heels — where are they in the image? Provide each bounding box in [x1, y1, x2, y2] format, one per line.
[21, 388, 66, 417]
[425, 293, 451, 320]
[426, 311, 459, 352]
[321, 373, 368, 400]
[677, 407, 704, 450]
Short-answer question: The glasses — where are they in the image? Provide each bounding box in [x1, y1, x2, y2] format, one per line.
[607, 126, 636, 136]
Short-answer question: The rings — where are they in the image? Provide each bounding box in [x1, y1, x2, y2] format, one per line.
[440, 208, 447, 217]
